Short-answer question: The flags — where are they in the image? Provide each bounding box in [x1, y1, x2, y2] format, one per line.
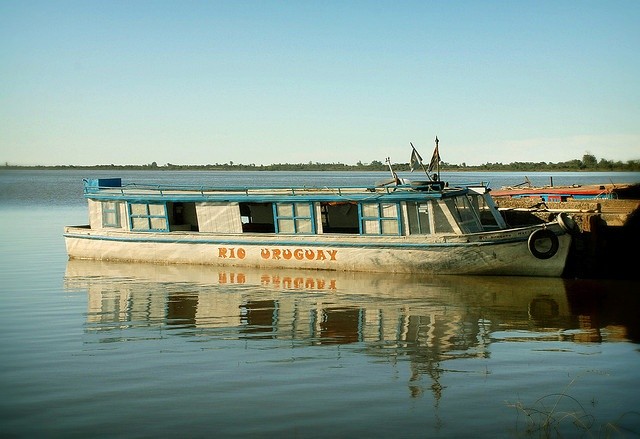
[410, 148, 423, 173]
[427, 147, 442, 174]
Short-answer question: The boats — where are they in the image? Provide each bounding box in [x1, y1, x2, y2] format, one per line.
[85, 275, 575, 401]
[486, 179, 638, 202]
[64, 136, 576, 273]
